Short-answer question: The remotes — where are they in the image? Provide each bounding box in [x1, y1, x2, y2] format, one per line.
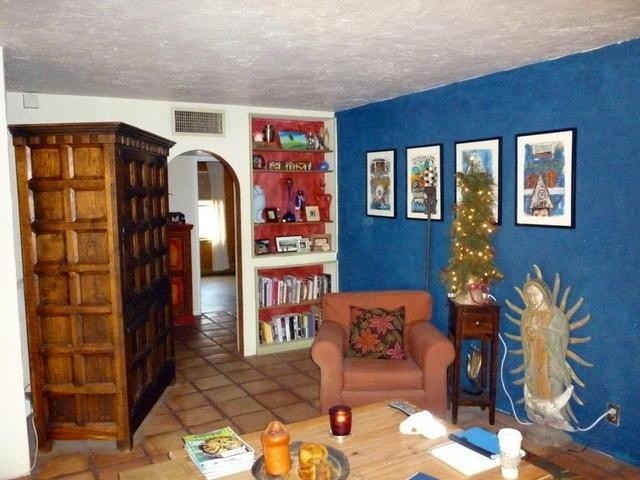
[389, 399, 418, 418]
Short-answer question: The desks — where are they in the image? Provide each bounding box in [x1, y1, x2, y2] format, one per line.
[117, 398, 569, 480]
[448, 299, 500, 426]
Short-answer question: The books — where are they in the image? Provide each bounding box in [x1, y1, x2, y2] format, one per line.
[182, 426, 256, 480]
[407, 471, 441, 480]
[447, 425, 504, 459]
[258, 304, 323, 345]
[258, 272, 331, 308]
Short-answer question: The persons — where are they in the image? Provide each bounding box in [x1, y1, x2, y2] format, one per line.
[295, 186, 306, 218]
[520, 278, 571, 432]
[530, 207, 550, 217]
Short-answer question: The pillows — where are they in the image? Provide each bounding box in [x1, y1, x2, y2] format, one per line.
[344, 304, 406, 363]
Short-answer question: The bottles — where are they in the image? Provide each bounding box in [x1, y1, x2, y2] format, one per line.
[329, 404, 352, 436]
[263, 125, 275, 142]
[260, 420, 292, 477]
[295, 188, 305, 222]
[318, 127, 323, 150]
[323, 128, 329, 150]
[306, 131, 315, 149]
[497, 428, 526, 480]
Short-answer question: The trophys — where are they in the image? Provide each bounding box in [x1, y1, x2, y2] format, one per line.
[282, 177, 296, 222]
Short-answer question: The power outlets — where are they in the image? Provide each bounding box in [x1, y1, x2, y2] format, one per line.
[607, 405, 619, 425]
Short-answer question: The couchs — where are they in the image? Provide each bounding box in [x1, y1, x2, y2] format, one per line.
[311, 290, 456, 417]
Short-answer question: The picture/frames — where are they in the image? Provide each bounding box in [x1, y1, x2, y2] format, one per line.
[405, 145, 443, 221]
[515, 127, 577, 229]
[453, 136, 503, 225]
[366, 149, 396, 219]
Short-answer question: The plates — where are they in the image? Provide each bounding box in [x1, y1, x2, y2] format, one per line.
[251, 441, 350, 480]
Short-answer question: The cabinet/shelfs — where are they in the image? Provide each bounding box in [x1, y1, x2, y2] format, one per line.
[248, 106, 339, 357]
[169, 222, 196, 327]
[8, 122, 177, 454]
